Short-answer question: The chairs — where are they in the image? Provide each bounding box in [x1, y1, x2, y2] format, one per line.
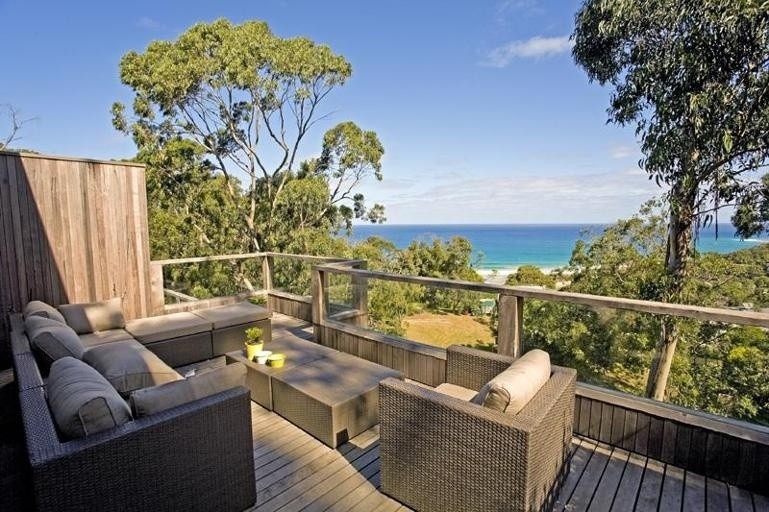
[374, 345, 580, 512]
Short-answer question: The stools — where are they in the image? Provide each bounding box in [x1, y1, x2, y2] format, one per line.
[125, 299, 274, 369]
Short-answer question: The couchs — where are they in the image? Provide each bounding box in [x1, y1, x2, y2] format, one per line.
[6, 297, 259, 512]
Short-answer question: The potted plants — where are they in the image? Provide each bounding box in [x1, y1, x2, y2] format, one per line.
[244, 326, 264, 361]
